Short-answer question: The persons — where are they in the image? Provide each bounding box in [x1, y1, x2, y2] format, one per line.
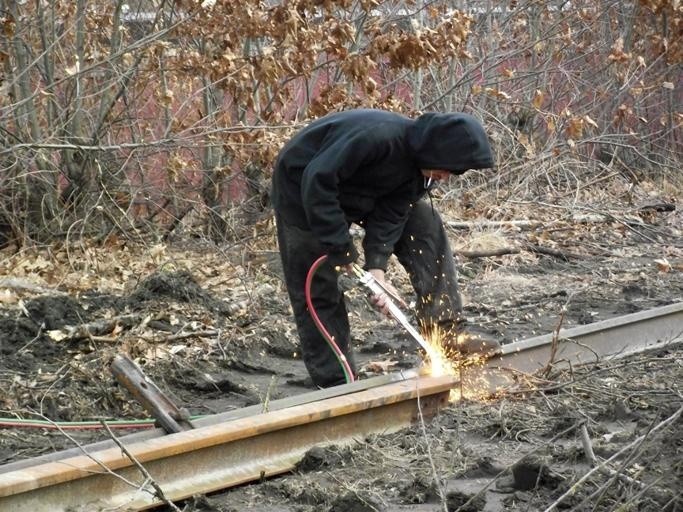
[270, 104, 501, 390]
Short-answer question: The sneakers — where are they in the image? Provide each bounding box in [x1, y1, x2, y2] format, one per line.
[445, 327, 500, 358]
